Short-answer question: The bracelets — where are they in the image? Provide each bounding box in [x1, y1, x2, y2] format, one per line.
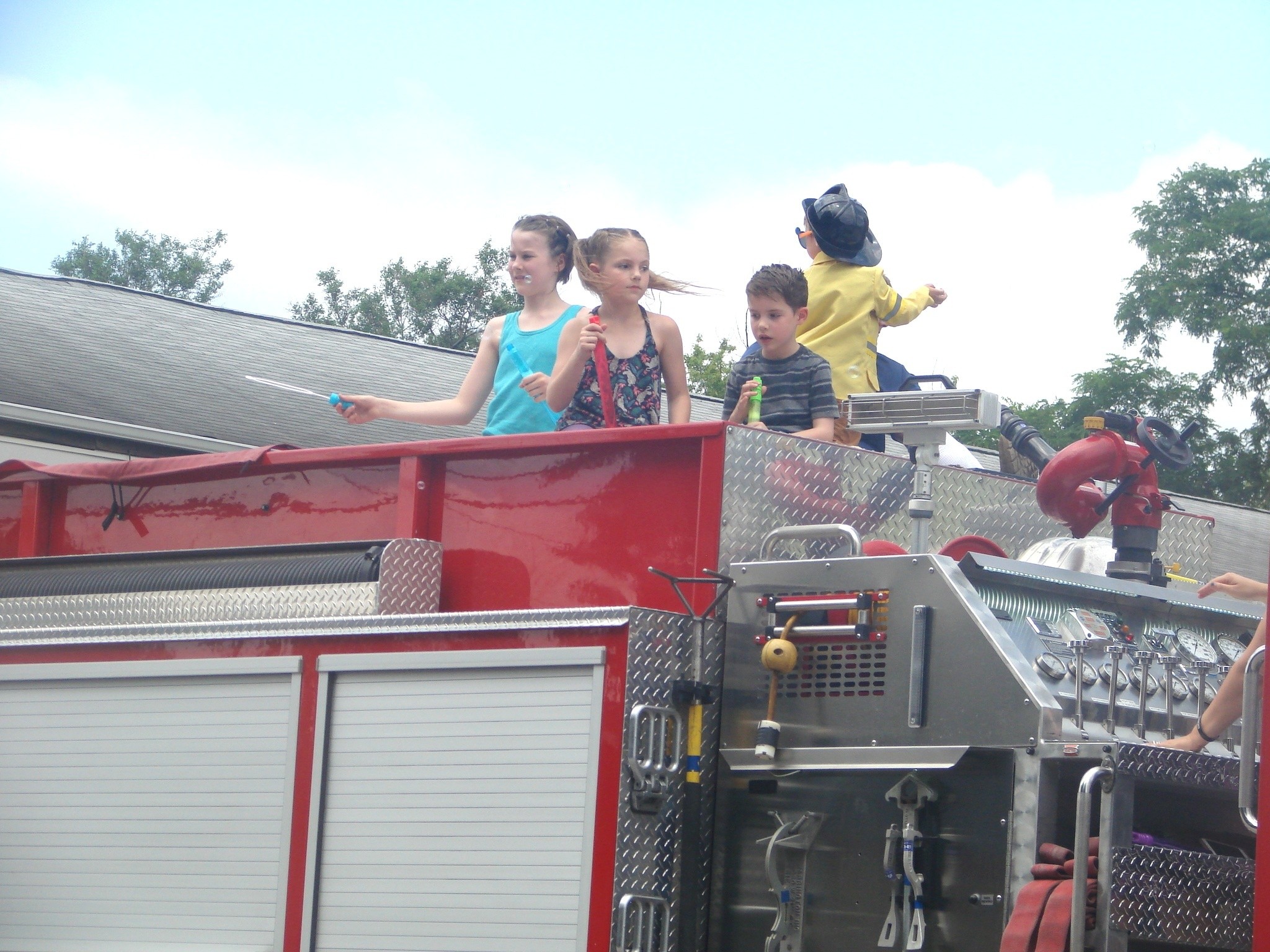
[1197, 715, 1220, 742]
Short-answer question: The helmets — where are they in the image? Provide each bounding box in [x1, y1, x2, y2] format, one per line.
[801, 184, 882, 267]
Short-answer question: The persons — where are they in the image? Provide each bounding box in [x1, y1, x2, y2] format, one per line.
[334, 215, 590, 437]
[794, 183, 947, 446]
[1149, 573, 1268, 752]
[720, 264, 840, 442]
[546, 228, 691, 433]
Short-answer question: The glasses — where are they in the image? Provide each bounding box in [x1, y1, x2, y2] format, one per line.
[795, 227, 813, 250]
